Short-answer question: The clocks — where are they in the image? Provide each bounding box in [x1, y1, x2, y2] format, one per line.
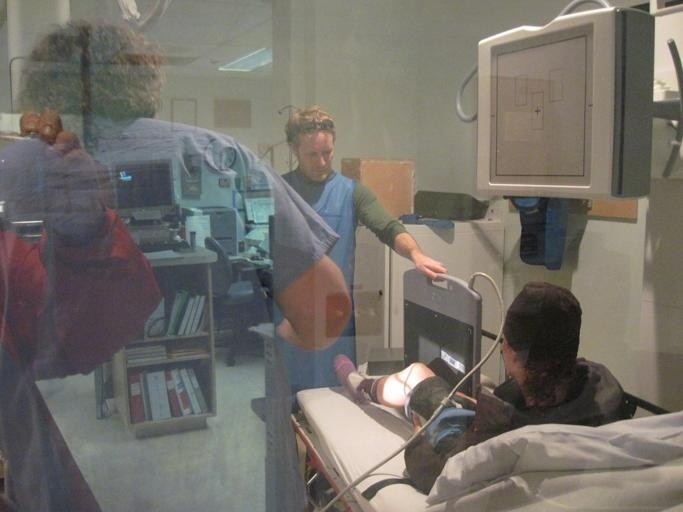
[204, 138, 237, 172]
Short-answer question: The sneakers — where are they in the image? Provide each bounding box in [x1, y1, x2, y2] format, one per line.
[331, 354, 366, 404]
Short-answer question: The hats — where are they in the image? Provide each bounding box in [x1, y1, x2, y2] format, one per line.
[502, 282, 583, 367]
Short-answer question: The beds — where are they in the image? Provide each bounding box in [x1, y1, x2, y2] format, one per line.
[292, 373, 681, 512]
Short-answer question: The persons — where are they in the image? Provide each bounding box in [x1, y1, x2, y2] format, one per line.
[332, 282, 628, 494]
[272, 102, 449, 503]
[0, 106, 164, 511]
[1, 13, 355, 512]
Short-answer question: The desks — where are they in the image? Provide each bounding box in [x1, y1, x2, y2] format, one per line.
[240, 251, 272, 278]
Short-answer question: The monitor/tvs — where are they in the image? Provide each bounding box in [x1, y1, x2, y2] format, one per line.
[108, 158, 178, 221]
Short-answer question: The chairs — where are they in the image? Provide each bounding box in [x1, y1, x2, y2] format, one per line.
[204, 236, 269, 367]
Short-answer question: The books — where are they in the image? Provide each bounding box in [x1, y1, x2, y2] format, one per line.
[127, 284, 212, 426]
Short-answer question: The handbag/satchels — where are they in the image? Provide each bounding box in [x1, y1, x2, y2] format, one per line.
[413, 191, 488, 222]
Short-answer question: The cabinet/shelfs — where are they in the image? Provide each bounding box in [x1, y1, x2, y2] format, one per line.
[113, 264, 219, 425]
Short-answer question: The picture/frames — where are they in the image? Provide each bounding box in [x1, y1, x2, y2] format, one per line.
[171, 98, 198, 132]
